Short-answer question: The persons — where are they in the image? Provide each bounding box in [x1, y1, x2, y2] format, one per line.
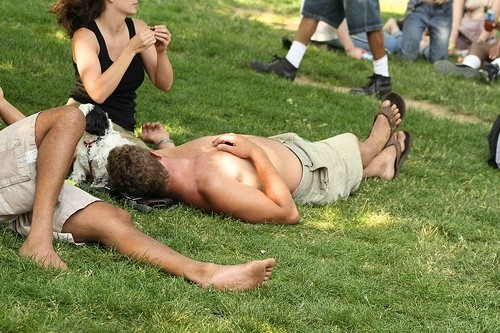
[487, 114, 500, 171]
[48, 0, 173, 170]
[300, 0, 401, 61]
[382, 0, 453, 63]
[105, 91, 411, 224]
[249, 0, 392, 100]
[434, 0, 500, 84]
[0, 87, 275, 292]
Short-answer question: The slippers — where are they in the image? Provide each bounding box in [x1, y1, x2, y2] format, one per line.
[382, 130, 412, 179]
[365, 91, 407, 149]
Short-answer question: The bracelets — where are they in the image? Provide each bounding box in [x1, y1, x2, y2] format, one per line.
[448, 38, 456, 49]
[156, 139, 173, 148]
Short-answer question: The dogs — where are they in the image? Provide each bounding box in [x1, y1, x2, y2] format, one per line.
[67, 102, 137, 190]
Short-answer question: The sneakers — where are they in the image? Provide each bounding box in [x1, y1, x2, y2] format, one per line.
[252, 55, 297, 82]
[432, 60, 480, 80]
[349, 73, 393, 97]
[478, 63, 500, 85]
